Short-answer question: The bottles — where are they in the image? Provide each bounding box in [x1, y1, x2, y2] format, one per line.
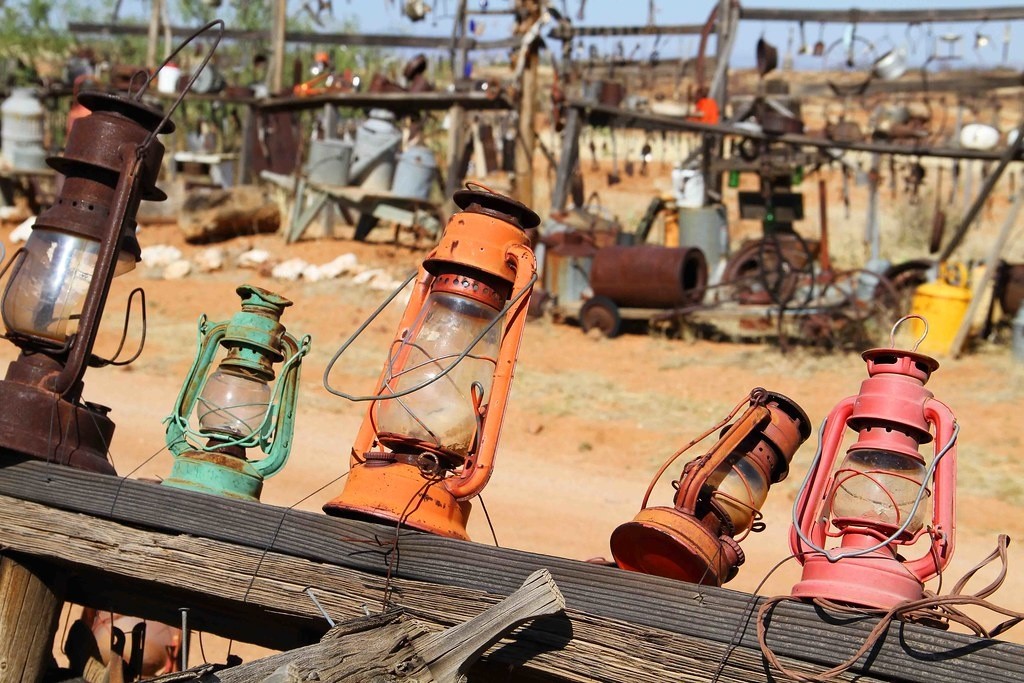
[790, 145, 802, 184]
[727, 142, 741, 188]
[765, 182, 777, 223]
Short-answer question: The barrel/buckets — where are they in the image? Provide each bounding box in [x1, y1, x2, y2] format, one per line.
[300, 139, 354, 187]
[671, 167, 705, 207]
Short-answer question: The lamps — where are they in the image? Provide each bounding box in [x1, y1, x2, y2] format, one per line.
[610, 386, 811, 590]
[786, 316, 959, 613]
[159, 283, 310, 503]
[319, 181, 544, 547]
[0, 19, 225, 480]
